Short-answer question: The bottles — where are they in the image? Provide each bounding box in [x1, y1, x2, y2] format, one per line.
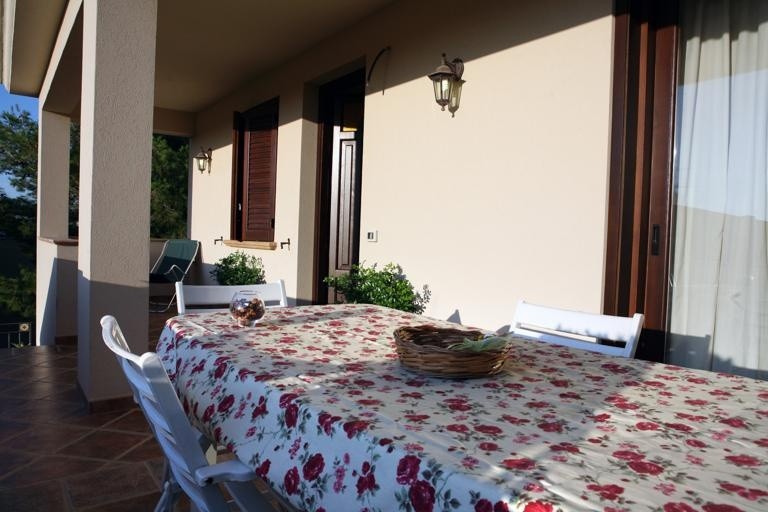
[231, 290, 265, 329]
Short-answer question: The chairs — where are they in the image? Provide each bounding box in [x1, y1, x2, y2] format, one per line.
[175, 279, 287, 315]
[100, 314, 292, 511]
[149, 239, 199, 313]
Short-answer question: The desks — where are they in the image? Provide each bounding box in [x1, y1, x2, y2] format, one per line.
[165, 301, 768, 511]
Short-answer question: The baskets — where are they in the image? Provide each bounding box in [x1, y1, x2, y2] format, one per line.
[392, 326, 512, 380]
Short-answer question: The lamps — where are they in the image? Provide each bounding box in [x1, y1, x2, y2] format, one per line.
[193, 145, 212, 174]
[428, 52, 464, 112]
